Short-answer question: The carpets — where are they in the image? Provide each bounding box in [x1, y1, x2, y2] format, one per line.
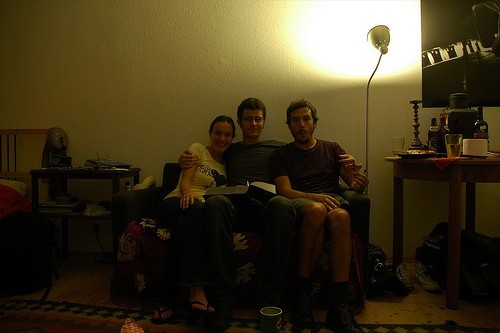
[0, 297, 499, 333]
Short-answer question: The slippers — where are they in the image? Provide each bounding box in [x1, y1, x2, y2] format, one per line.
[184, 299, 216, 316]
[151, 306, 174, 324]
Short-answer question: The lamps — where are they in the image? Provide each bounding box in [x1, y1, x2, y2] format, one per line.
[365, 25, 390, 195]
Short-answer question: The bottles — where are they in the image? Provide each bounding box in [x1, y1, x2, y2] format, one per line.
[473, 105, 489, 139]
[437, 113, 448, 153]
[427, 117, 438, 150]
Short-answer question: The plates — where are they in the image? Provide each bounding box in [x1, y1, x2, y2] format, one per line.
[397, 150, 436, 158]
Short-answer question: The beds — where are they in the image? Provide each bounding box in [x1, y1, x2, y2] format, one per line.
[0, 129, 49, 297]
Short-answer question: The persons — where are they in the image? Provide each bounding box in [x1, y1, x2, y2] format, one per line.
[178, 96, 364, 331]
[150, 113, 237, 324]
[269, 98, 371, 332]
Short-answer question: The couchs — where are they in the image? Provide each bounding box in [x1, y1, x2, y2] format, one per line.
[111, 163, 370, 301]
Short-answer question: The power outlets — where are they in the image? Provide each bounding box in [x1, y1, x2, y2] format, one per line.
[91, 220, 103, 232]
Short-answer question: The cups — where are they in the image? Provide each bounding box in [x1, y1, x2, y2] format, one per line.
[96, 151, 109, 170]
[392, 138, 405, 156]
[444, 133, 463, 159]
[260, 307, 282, 333]
[463, 139, 488, 156]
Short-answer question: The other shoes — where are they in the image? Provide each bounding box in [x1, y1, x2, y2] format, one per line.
[205, 298, 234, 332]
[395, 264, 414, 291]
[326, 302, 365, 333]
[288, 291, 317, 329]
[414, 262, 442, 293]
[256, 292, 282, 317]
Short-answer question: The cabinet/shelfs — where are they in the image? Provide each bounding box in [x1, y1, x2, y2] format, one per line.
[29, 167, 142, 252]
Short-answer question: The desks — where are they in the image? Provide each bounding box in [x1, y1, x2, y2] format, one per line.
[385, 157, 500, 310]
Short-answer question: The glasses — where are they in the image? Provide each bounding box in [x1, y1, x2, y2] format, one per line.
[241, 118, 263, 123]
